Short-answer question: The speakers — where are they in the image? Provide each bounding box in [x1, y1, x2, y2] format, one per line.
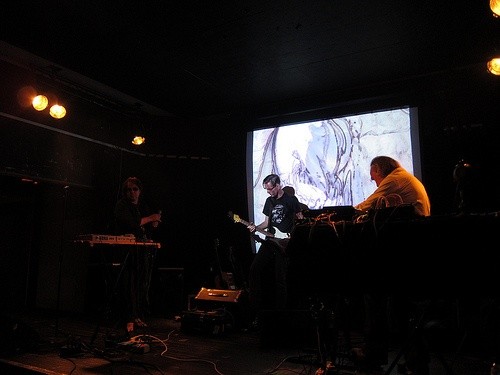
[194, 287, 251, 314]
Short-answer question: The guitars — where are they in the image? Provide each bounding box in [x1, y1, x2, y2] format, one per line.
[230, 212, 294, 242]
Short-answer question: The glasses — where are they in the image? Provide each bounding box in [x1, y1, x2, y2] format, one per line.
[127, 187, 139, 192]
[264, 187, 275, 190]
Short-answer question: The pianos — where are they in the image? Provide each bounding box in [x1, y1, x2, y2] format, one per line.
[67, 234, 163, 356]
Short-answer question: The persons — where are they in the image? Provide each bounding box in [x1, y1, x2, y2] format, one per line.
[115, 177, 161, 326]
[353, 156, 432, 217]
[247, 174, 300, 304]
